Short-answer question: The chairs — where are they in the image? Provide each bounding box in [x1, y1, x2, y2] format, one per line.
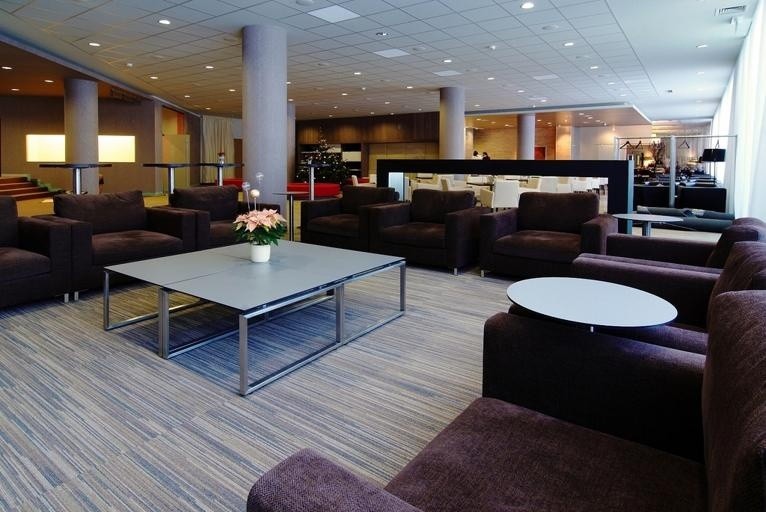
[349, 166, 609, 214]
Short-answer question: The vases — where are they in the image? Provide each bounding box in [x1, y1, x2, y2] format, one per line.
[248, 240, 274, 264]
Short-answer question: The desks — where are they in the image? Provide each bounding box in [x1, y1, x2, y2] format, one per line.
[611, 210, 687, 239]
[498, 272, 678, 337]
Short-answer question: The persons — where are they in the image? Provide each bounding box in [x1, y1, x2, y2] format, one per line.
[482, 152, 490, 160]
[471, 151, 483, 160]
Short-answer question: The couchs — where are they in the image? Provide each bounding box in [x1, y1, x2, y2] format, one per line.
[146, 185, 250, 245]
[298, 185, 398, 254]
[630, 171, 734, 232]
[29, 188, 197, 302]
[369, 188, 481, 277]
[569, 215, 761, 359]
[480, 192, 611, 279]
[241, 288, 761, 512]
[0, 189, 75, 311]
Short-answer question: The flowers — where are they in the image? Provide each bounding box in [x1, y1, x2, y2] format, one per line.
[231, 207, 287, 247]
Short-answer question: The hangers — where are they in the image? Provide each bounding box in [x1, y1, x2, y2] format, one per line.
[618, 135, 722, 150]
